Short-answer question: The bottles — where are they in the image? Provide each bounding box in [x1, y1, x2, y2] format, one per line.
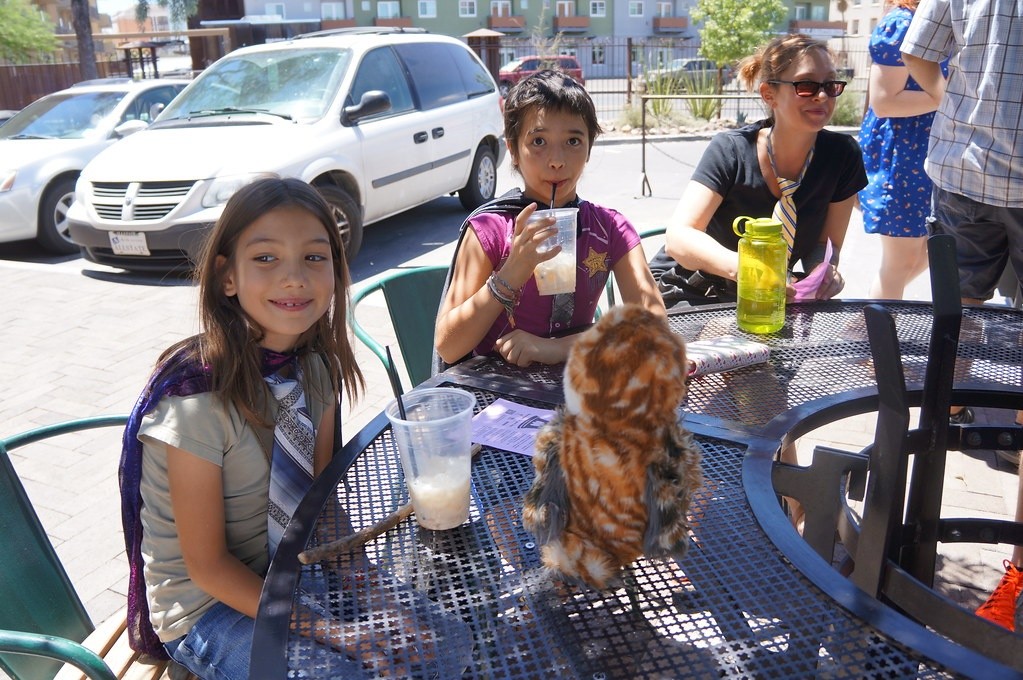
[732, 216, 787, 334]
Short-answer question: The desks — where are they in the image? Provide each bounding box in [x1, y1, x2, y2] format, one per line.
[247, 301, 1023, 680]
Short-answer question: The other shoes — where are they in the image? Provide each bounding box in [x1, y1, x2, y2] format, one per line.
[791, 502, 804, 527]
[949, 407, 975, 425]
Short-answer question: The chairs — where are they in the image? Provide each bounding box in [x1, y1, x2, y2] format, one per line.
[0, 223, 812, 680]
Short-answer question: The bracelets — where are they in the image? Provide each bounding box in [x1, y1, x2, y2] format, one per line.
[341, 566, 382, 598]
[486, 271, 523, 328]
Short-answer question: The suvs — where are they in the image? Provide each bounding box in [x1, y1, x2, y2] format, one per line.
[499, 55, 584, 97]
[638, 57, 732, 89]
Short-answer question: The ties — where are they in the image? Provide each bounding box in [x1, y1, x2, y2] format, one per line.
[767, 124, 815, 269]
[254, 351, 328, 617]
[549, 291, 575, 339]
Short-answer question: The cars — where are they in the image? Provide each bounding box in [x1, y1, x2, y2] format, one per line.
[0, 80, 240, 255]
[0, 75, 143, 128]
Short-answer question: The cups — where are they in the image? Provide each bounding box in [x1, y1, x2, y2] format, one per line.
[384, 388, 476, 530]
[526, 208, 580, 295]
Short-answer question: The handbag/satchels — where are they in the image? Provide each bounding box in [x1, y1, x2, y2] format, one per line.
[647, 244, 722, 344]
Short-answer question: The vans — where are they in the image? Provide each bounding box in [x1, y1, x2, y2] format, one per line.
[64, 33, 507, 273]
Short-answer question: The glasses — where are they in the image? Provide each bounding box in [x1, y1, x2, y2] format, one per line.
[762, 80, 847, 98]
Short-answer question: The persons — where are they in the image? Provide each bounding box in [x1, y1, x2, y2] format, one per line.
[138, 176, 475, 680]
[648, 33, 868, 579]
[432, 69, 669, 680]
[842, 0, 1023, 630]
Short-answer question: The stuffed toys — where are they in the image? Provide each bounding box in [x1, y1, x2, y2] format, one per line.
[522, 301, 700, 588]
